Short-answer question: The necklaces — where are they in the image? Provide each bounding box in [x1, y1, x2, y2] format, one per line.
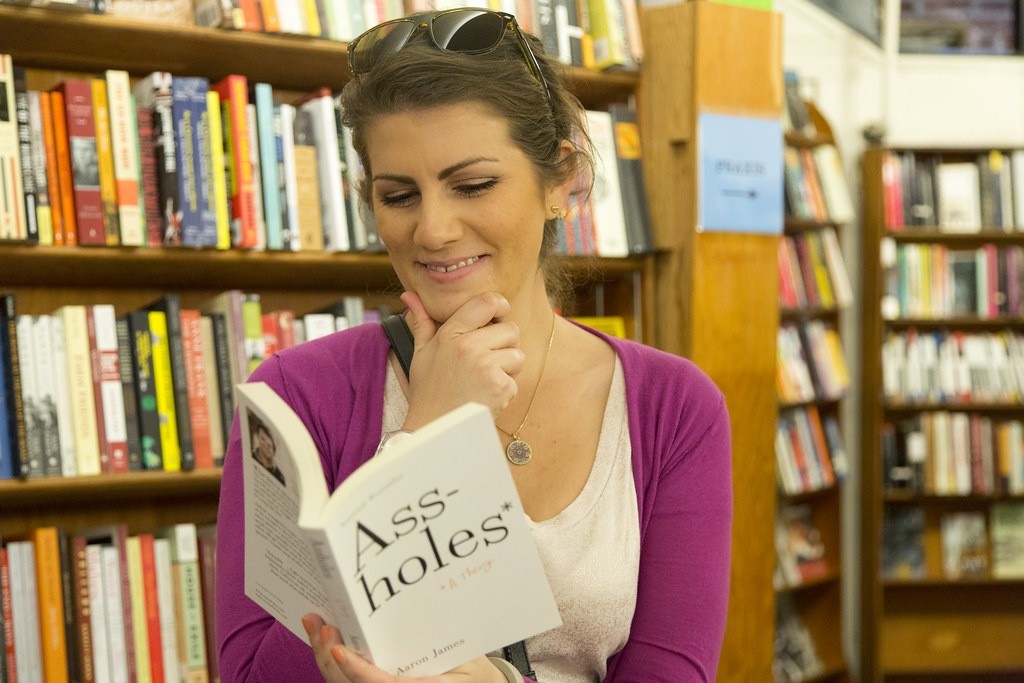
[481, 306, 562, 467]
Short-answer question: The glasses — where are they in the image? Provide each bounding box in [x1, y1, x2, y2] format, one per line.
[347, 8, 564, 143]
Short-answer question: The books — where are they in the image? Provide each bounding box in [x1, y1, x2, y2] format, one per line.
[0, 0, 648, 68]
[0, 48, 658, 683]
[754, 63, 1024, 681]
[235, 379, 565, 683]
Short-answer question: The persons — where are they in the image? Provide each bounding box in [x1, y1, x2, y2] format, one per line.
[213, 5, 739, 683]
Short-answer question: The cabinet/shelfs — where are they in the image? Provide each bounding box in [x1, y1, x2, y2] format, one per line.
[2, 0, 678, 681]
[858, 125, 1023, 681]
[767, 98, 857, 683]
